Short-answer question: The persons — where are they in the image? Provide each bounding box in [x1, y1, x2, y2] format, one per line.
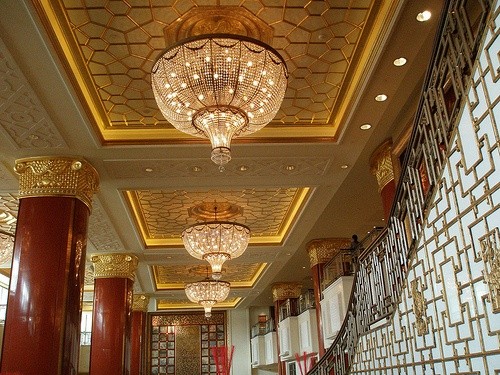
[349, 234, 362, 274]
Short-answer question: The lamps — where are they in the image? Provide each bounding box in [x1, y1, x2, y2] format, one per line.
[181, 199, 252, 274]
[184, 266, 232, 319]
[149, 36, 290, 168]
